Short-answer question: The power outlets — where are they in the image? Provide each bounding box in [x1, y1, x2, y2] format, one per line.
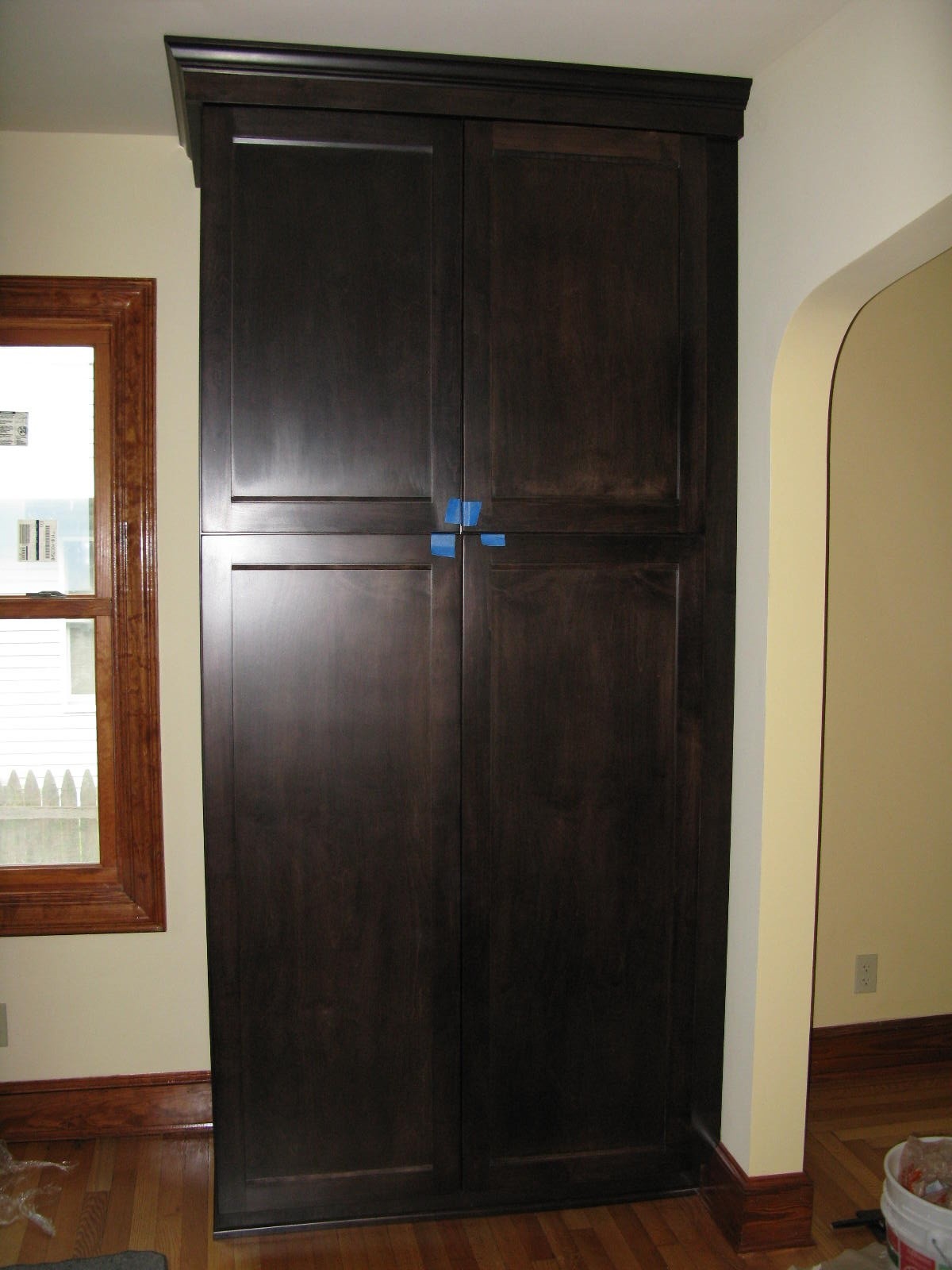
[854, 954, 878, 993]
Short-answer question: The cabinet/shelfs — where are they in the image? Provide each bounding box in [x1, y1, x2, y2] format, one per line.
[162, 33, 752, 1238]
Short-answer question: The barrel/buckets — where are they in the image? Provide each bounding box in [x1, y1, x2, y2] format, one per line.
[880, 1137, 952, 1270]
